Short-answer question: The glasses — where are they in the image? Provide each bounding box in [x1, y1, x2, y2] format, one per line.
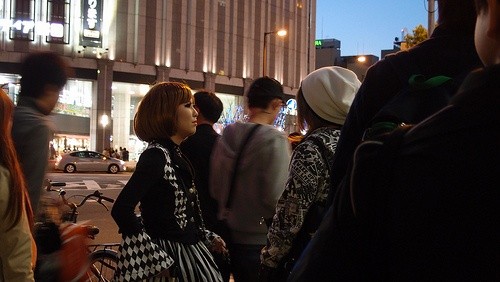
[281, 100, 287, 107]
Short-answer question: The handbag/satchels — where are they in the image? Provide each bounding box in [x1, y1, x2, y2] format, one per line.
[208, 218, 234, 282]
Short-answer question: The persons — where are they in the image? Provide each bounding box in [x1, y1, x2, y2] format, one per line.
[10, 50, 63, 281]
[47, 142, 129, 160]
[0, 89, 34, 282]
[260, 0, 500, 282]
[209, 77, 290, 282]
[110, 83, 230, 282]
[178, 90, 230, 282]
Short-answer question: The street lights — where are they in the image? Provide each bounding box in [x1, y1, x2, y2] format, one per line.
[263, 30, 287, 78]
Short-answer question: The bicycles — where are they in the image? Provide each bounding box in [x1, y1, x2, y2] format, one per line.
[47, 182, 123, 282]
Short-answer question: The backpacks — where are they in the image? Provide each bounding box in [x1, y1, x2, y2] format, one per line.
[364, 50, 456, 146]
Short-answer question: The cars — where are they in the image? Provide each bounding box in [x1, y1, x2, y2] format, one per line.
[54, 150, 127, 174]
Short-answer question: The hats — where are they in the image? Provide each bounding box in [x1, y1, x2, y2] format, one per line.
[301, 66, 362, 124]
[246, 75, 296, 103]
